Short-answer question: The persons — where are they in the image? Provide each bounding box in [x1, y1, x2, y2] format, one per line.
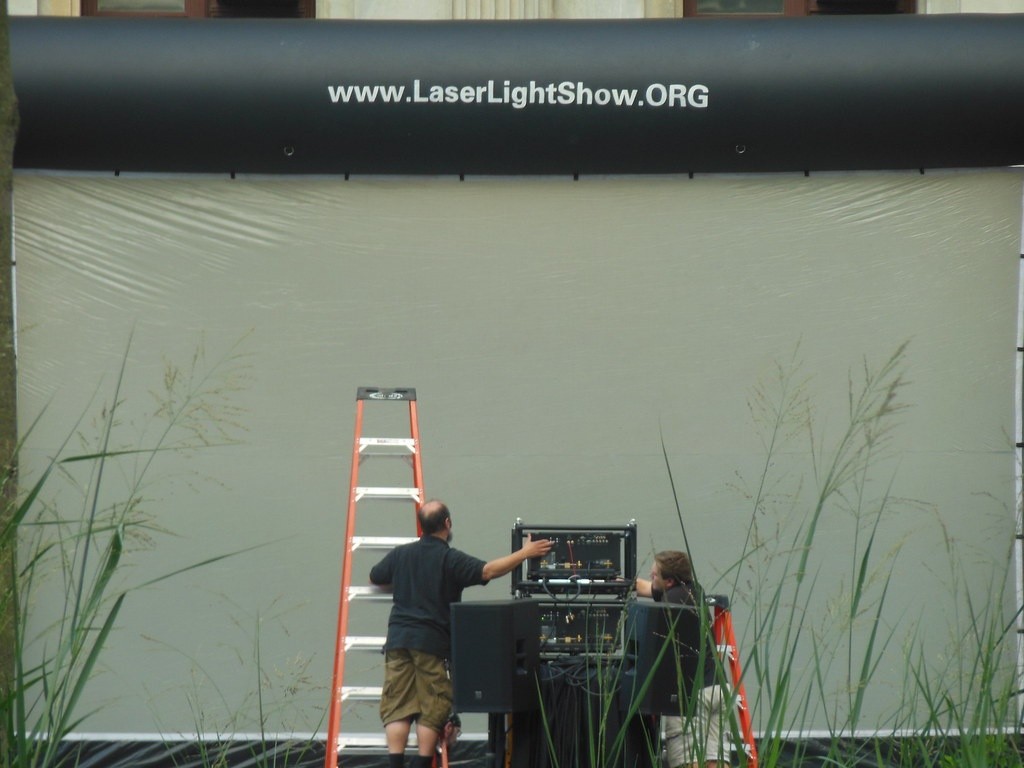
[637, 550, 731, 768]
[369, 501, 555, 768]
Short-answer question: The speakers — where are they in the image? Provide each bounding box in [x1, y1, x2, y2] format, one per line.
[448, 600, 545, 714]
[619, 601, 703, 716]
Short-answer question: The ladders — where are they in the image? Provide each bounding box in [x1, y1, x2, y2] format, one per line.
[324, 383, 450, 768]
[704, 591, 759, 768]
